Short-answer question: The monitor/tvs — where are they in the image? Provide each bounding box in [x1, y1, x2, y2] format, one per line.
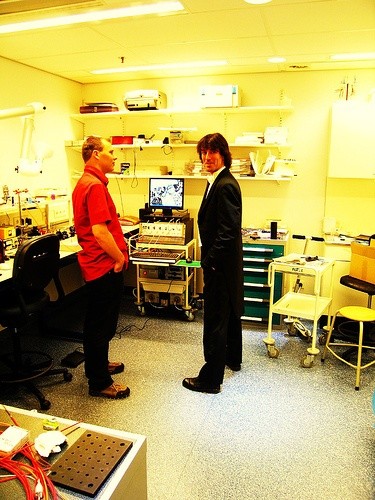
[148, 177, 185, 215]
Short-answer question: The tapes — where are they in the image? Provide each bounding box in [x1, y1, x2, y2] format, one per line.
[160, 166, 167, 171]
[161, 171, 167, 175]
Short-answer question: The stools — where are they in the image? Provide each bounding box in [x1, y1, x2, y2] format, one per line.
[321, 305, 375, 391]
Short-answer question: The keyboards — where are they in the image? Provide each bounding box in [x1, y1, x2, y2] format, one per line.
[131, 252, 179, 259]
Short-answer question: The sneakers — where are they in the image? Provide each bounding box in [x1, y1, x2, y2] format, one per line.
[89, 381, 130, 399]
[108, 362, 124, 375]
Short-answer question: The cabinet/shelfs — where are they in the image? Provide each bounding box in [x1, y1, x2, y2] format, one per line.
[241, 232, 289, 330]
[72, 106, 293, 181]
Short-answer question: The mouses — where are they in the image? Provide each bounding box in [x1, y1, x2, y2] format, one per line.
[186, 258, 192, 263]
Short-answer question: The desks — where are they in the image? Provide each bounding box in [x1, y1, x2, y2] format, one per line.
[0, 223, 139, 289]
[0, 404, 147, 500]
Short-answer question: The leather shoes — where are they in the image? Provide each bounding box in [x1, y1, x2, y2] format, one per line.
[226, 361, 241, 371]
[183, 378, 220, 394]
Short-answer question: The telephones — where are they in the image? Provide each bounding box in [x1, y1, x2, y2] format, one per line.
[118, 216, 141, 226]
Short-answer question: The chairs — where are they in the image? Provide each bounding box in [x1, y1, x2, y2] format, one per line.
[0, 233, 72, 410]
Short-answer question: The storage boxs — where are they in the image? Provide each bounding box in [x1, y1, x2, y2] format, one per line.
[112, 136, 135, 144]
[350, 242, 375, 285]
[200, 85, 241, 108]
[80, 106, 119, 114]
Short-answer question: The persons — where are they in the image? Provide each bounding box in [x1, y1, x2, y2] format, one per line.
[71, 135, 133, 399]
[182, 134, 244, 394]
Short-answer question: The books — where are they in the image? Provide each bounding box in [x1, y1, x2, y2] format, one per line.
[184, 158, 252, 177]
